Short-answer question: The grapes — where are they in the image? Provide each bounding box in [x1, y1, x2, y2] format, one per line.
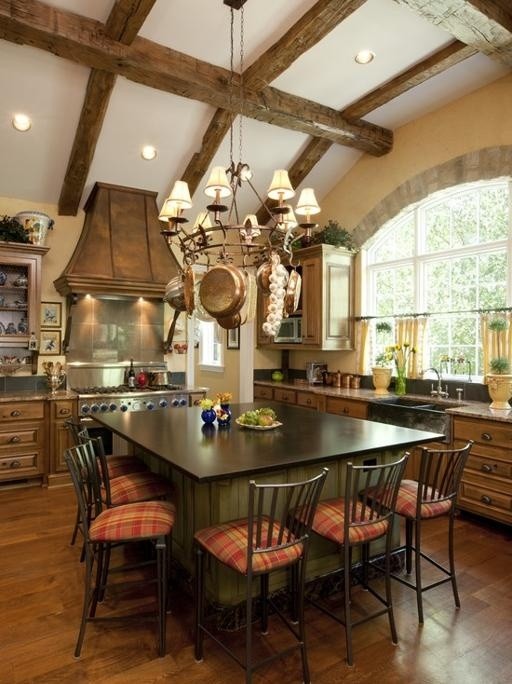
[241, 407, 276, 424]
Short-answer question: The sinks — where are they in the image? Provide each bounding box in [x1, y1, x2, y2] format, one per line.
[370, 395, 435, 409]
[412, 401, 466, 414]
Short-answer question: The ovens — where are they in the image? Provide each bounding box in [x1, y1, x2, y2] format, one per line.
[55, 418, 130, 473]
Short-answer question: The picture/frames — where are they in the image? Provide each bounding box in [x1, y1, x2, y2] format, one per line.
[41, 302, 62, 328]
[227, 326, 239, 349]
[40, 330, 62, 356]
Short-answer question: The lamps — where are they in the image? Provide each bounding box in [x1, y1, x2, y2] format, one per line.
[160, 1, 324, 350]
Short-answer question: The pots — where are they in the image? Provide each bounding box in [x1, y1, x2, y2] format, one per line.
[149, 369, 172, 386]
[216, 312, 242, 330]
[162, 272, 187, 312]
[283, 269, 303, 319]
[199, 263, 247, 319]
[255, 263, 271, 295]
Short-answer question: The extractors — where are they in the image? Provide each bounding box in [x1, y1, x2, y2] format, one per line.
[53, 182, 184, 301]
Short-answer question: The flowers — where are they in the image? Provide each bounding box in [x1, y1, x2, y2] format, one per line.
[199, 398, 217, 411]
[384, 343, 416, 375]
[216, 392, 231, 404]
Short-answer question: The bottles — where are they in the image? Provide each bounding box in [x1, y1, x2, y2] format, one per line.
[128, 359, 136, 389]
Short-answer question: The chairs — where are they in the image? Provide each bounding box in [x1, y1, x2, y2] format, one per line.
[288, 452, 412, 667]
[58, 429, 176, 661]
[190, 467, 331, 682]
[356, 439, 475, 627]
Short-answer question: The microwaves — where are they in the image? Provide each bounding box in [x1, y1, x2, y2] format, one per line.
[273, 317, 302, 344]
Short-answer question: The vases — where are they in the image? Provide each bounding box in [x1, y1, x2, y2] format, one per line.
[218, 403, 231, 426]
[395, 358, 407, 394]
[201, 405, 216, 423]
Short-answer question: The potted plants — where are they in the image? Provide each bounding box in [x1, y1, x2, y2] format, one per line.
[373, 322, 392, 395]
[486, 317, 512, 409]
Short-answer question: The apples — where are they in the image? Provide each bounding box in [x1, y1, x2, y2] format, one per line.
[257, 416, 273, 425]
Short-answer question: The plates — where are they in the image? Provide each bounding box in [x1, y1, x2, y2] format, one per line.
[235, 418, 281, 430]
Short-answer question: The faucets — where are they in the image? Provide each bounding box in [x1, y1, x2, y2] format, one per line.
[419, 368, 442, 393]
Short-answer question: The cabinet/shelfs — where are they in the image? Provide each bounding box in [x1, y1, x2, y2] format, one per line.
[0, 240, 51, 349]
[0, 402, 45, 489]
[448, 415, 512, 527]
[257, 245, 357, 349]
[49, 401, 78, 475]
[254, 384, 369, 418]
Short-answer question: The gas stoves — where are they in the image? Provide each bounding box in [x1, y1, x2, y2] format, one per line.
[65, 382, 207, 418]
